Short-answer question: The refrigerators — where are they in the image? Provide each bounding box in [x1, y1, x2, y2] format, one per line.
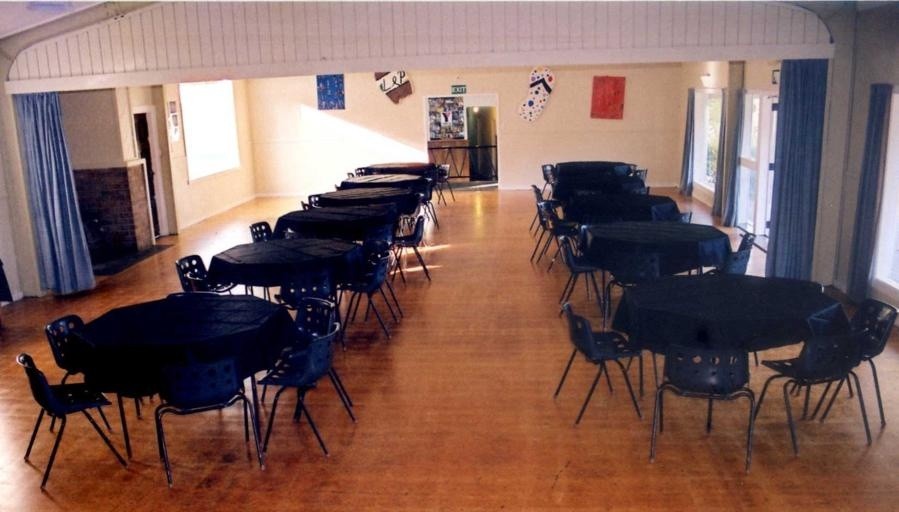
[467, 105, 498, 181]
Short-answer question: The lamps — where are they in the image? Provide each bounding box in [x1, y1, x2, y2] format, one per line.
[701, 73, 714, 90]
